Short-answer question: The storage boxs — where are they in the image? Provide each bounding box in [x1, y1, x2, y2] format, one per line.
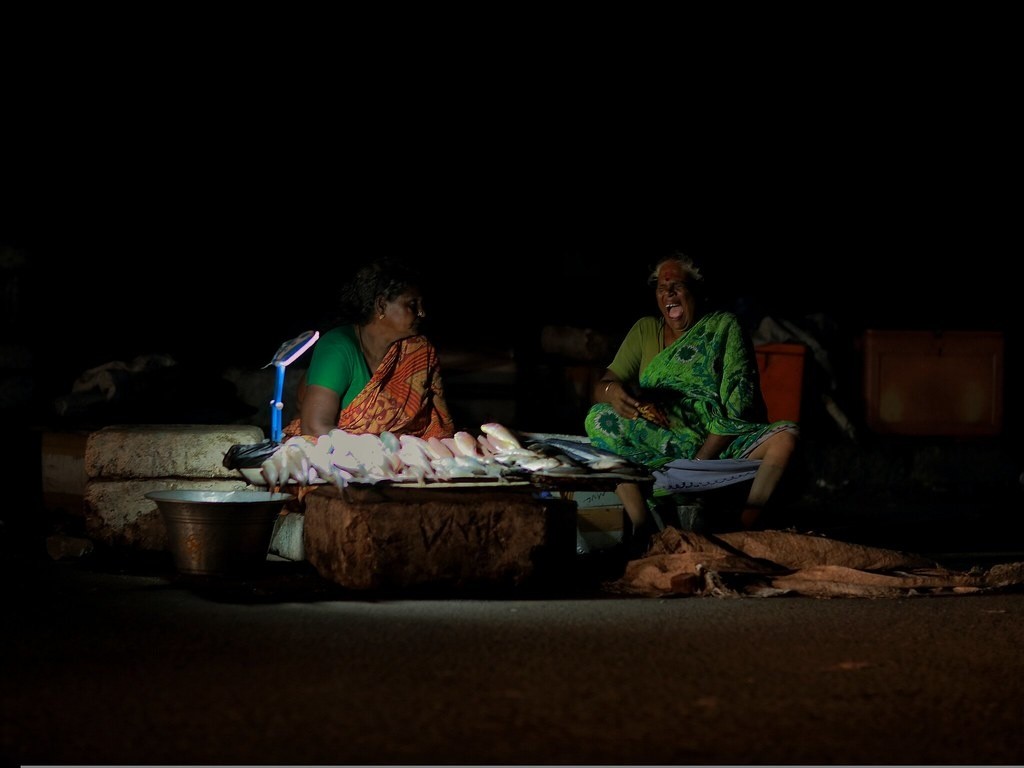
[748, 342, 806, 426]
[434, 348, 519, 424]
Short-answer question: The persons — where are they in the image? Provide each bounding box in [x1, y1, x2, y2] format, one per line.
[584, 256, 799, 544]
[267, 266, 455, 562]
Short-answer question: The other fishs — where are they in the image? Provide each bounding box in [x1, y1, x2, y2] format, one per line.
[261, 423, 627, 495]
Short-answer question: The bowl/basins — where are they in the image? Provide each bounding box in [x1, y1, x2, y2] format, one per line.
[146, 490, 292, 576]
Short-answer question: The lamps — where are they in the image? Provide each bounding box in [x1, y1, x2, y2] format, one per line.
[258, 327, 320, 487]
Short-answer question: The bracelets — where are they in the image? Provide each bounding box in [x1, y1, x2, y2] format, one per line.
[605, 382, 614, 396]
[695, 456, 701, 461]
